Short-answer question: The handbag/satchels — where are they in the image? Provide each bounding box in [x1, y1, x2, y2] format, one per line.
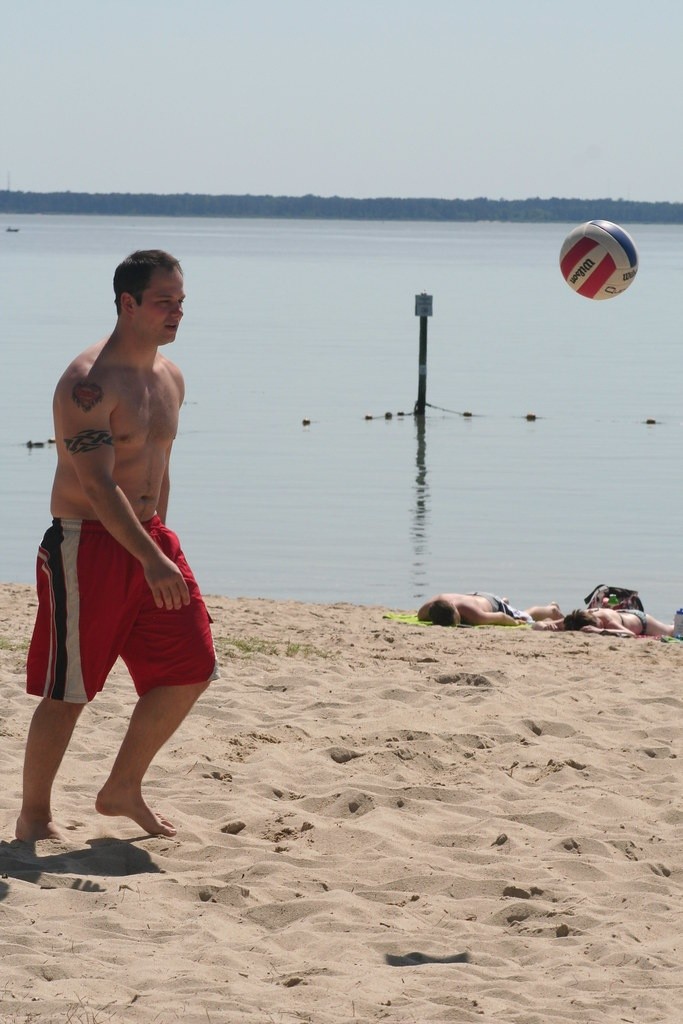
[584, 584, 643, 611]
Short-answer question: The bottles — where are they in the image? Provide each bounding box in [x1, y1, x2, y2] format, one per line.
[601, 594, 620, 609]
[672, 608, 683, 640]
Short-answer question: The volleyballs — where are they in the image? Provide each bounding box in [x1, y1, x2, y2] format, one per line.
[560, 220, 640, 300]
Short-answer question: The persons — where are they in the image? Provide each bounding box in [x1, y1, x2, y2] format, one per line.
[417, 590, 565, 627]
[531, 609, 674, 638]
[15, 250, 217, 840]
